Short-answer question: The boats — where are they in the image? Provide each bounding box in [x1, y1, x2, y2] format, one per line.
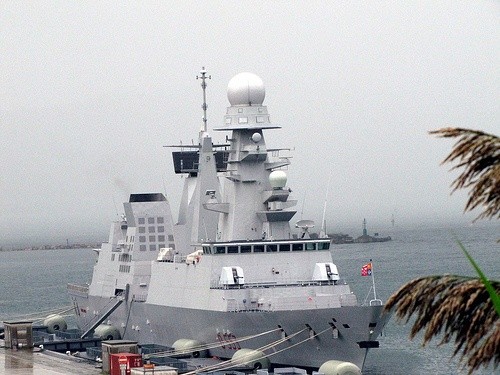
[290, 230, 353, 244]
[334, 217, 392, 244]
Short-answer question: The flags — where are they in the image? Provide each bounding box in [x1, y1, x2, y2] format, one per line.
[361, 263, 372, 277]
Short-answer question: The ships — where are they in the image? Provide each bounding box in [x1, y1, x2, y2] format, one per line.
[59, 67, 390, 372]
